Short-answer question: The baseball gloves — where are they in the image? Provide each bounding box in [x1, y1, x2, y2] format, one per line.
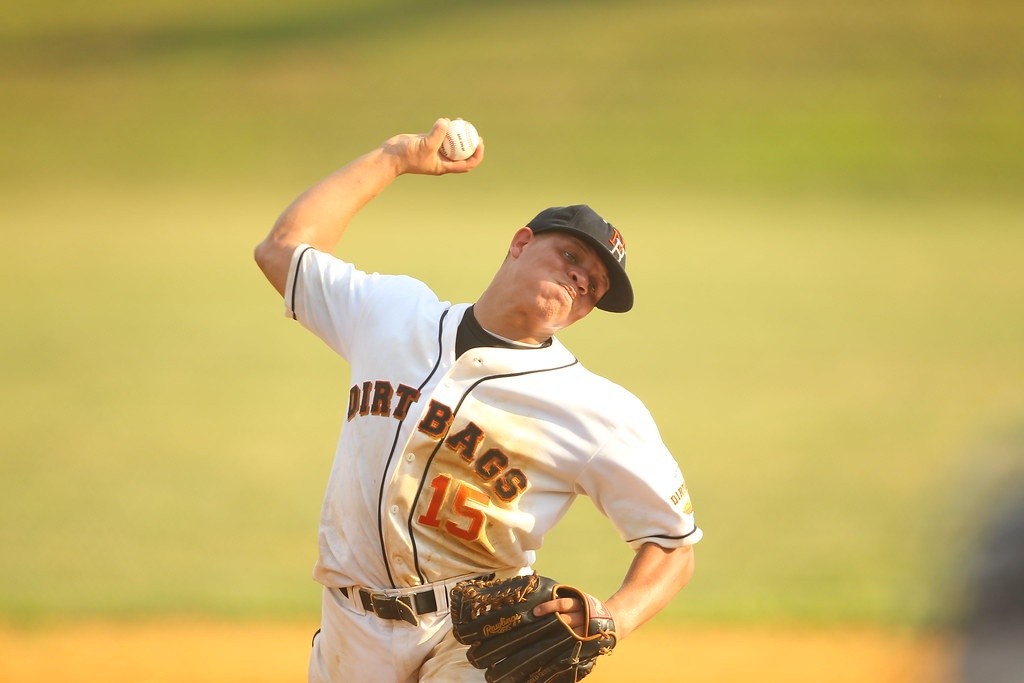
[448, 568, 618, 683]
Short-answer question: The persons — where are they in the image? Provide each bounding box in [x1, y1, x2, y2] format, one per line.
[255, 119, 703, 683]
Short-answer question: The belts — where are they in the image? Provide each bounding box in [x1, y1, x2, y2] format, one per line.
[338, 573, 497, 627]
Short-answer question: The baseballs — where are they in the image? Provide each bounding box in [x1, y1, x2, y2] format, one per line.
[439, 119, 480, 162]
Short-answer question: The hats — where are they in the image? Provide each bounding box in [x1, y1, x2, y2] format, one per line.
[528, 204, 634, 313]
